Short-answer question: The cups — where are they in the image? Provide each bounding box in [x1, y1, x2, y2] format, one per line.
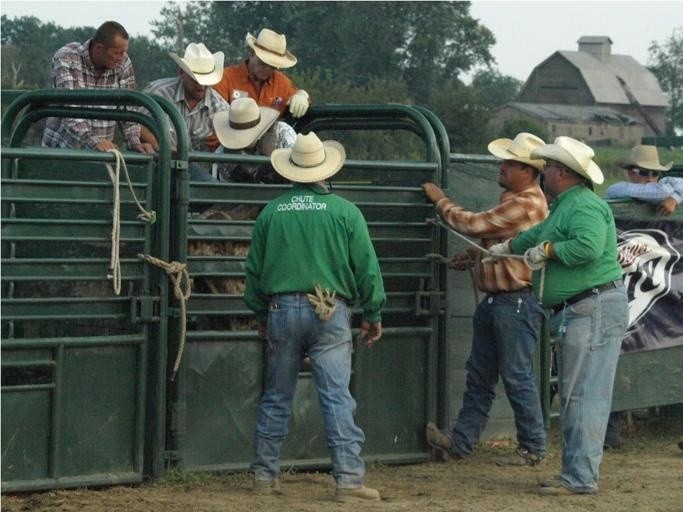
[632, 168, 661, 177]
[544, 162, 550, 170]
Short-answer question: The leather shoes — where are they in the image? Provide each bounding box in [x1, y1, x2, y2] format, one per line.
[314, 299, 336, 321]
[306, 284, 337, 305]
[480, 237, 513, 264]
[528, 240, 551, 264]
[287, 89, 310, 118]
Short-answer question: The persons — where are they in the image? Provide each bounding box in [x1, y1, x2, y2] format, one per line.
[128, 43, 230, 214]
[481, 135, 630, 494]
[41, 21, 156, 149]
[213, 29, 312, 118]
[425, 134, 547, 464]
[606, 144, 683, 215]
[210, 97, 296, 182]
[242, 130, 387, 503]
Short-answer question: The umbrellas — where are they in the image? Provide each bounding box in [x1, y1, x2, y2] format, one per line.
[604, 426, 626, 452]
[493, 451, 529, 468]
[425, 420, 457, 464]
[251, 477, 281, 495]
[535, 477, 576, 496]
[334, 484, 381, 505]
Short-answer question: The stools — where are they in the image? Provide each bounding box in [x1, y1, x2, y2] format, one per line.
[553, 283, 614, 313]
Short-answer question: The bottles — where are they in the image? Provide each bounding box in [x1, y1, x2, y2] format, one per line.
[169, 41, 225, 86]
[529, 136, 603, 185]
[487, 132, 546, 174]
[212, 96, 281, 151]
[613, 144, 673, 172]
[271, 130, 347, 184]
[245, 27, 298, 68]
